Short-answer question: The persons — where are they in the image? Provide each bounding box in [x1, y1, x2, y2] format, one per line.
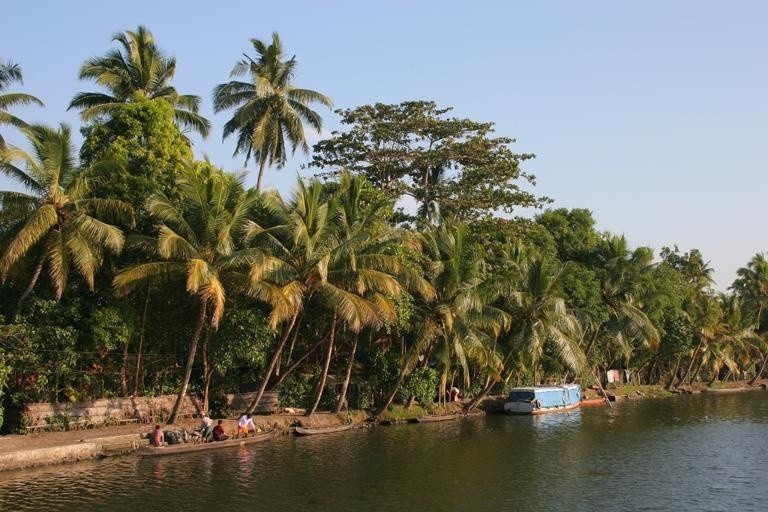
[213, 419, 234, 441]
[198, 411, 214, 443]
[446, 382, 461, 402]
[150, 424, 168, 447]
[236, 413, 256, 439]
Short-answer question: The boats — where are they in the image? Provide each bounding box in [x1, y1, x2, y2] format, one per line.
[294, 420, 356, 435]
[503, 383, 581, 414]
[504, 406, 581, 437]
[139, 422, 278, 455]
[415, 412, 459, 422]
